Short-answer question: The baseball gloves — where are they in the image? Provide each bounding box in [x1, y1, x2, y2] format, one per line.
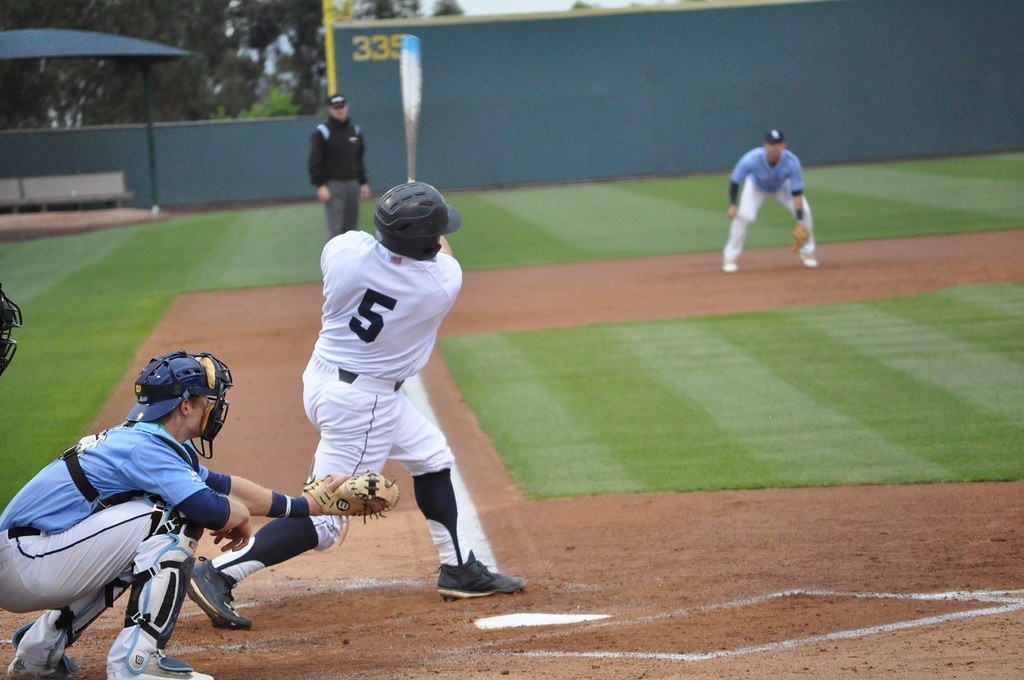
[790, 224, 811, 254]
[305, 469, 400, 517]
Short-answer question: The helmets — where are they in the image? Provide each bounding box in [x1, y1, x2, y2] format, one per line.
[125, 350, 222, 423]
[376, 182, 461, 260]
[0, 284, 22, 375]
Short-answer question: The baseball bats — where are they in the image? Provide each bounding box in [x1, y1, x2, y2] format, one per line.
[399, 33, 424, 184]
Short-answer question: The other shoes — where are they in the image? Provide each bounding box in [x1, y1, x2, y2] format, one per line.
[723, 258, 738, 274]
[801, 251, 819, 267]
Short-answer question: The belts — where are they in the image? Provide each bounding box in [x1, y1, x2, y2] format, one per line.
[338, 371, 405, 392]
[6, 527, 41, 539]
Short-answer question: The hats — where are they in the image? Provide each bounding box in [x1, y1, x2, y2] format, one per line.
[327, 94, 346, 108]
[766, 129, 784, 143]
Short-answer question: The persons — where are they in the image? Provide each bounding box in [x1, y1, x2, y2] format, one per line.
[0, 351, 404, 680]
[185, 182, 527, 628]
[309, 95, 370, 245]
[723, 130, 817, 272]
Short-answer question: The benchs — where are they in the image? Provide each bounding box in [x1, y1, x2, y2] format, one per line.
[0, 170, 135, 213]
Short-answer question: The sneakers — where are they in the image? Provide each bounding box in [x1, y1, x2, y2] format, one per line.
[437, 560, 526, 598]
[105, 647, 212, 680]
[185, 558, 253, 630]
[6, 653, 84, 680]
